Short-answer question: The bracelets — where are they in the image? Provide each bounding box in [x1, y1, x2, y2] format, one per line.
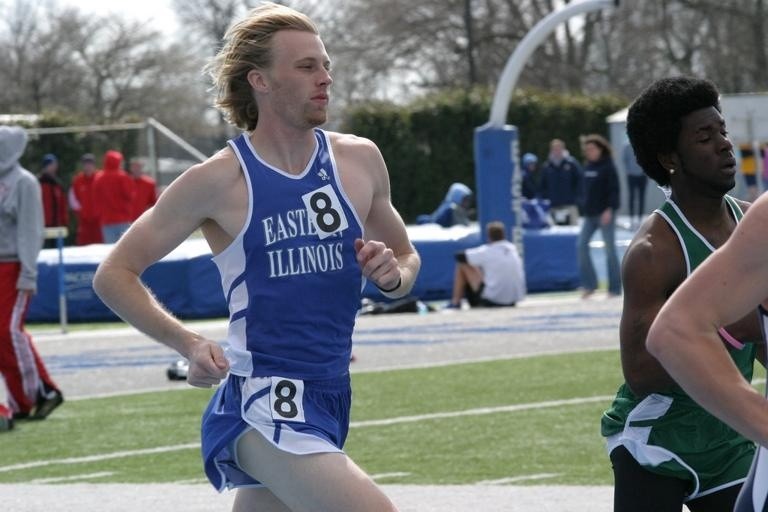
[371, 274, 402, 294]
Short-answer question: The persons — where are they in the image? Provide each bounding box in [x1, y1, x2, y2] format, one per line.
[621, 140, 646, 229]
[94, 6, 423, 512]
[599, 75, 766, 512]
[645, 189, 767, 511]
[521, 139, 588, 226]
[0, 123, 64, 434]
[429, 183, 473, 227]
[576, 134, 622, 302]
[38, 149, 158, 244]
[446, 221, 527, 308]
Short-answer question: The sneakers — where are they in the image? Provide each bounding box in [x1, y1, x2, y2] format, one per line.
[34, 392, 62, 420]
